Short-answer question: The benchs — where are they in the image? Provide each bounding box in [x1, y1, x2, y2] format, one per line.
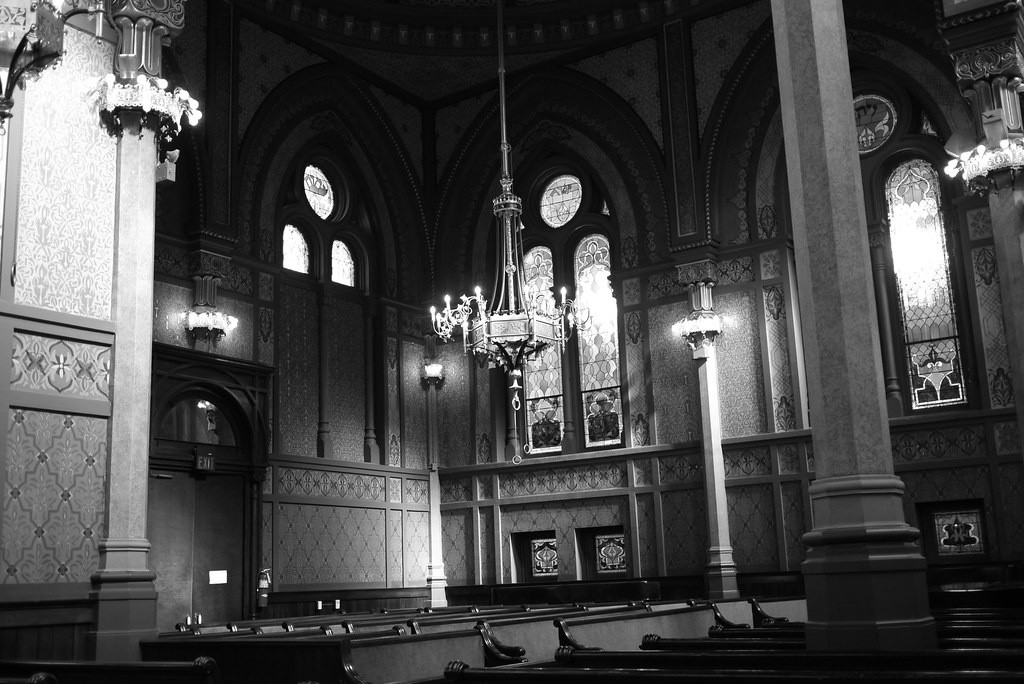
[0, 600, 1024, 684]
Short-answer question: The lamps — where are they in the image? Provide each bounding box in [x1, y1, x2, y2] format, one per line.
[100, 72, 202, 148]
[428, 0, 596, 411]
[943, 138, 1024, 200]
[184, 308, 239, 345]
[670, 312, 730, 351]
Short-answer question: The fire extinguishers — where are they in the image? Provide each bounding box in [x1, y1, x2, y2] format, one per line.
[257, 568, 271, 608]
